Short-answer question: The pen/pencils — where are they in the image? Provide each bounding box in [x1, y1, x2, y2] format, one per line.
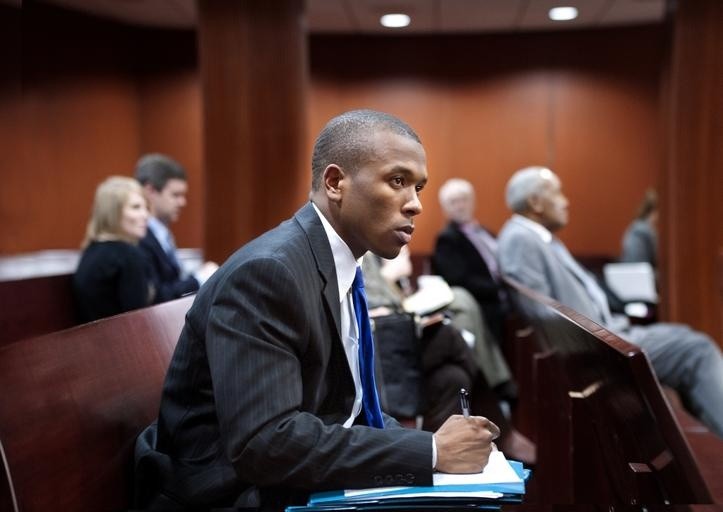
[457, 388, 471, 419]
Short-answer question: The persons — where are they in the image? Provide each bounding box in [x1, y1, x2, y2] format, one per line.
[620, 188, 659, 265]
[431, 177, 522, 360]
[360, 240, 536, 469]
[494, 164, 721, 435]
[72, 176, 156, 326]
[134, 108, 499, 510]
[135, 154, 223, 305]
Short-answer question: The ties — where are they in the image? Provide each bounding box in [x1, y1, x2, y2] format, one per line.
[351, 266, 385, 430]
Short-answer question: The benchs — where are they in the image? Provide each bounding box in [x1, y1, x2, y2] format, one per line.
[0, 274, 196, 512]
[500, 275, 722, 511]
